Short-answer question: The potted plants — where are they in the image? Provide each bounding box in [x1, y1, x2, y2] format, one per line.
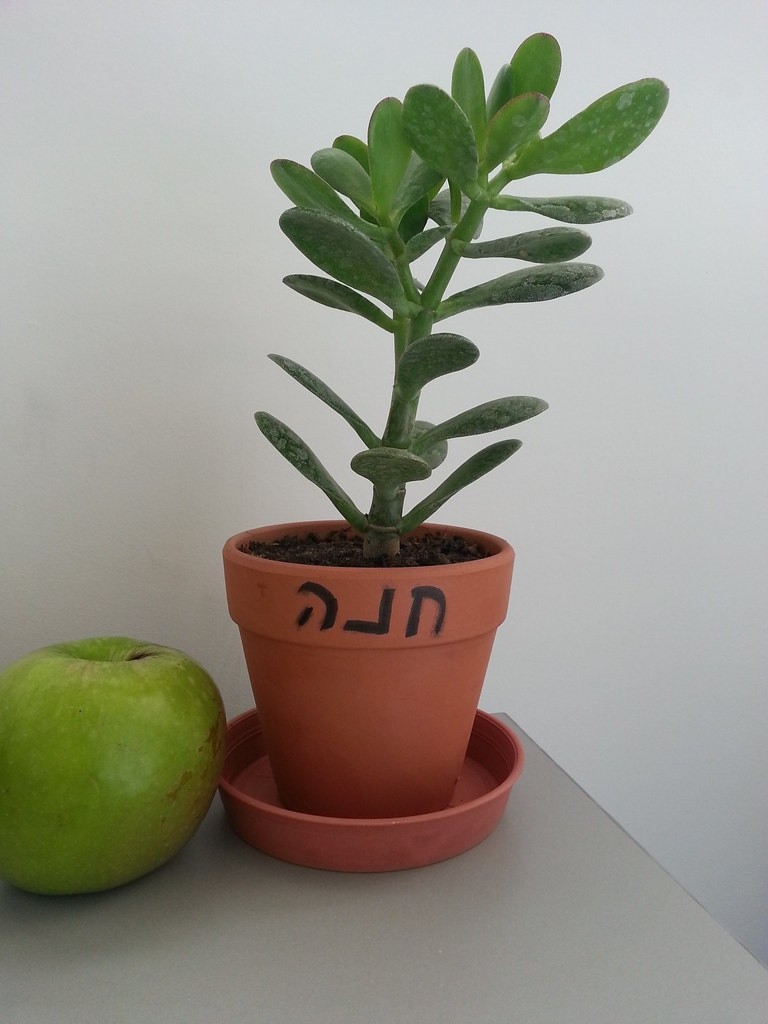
[218, 31, 671, 874]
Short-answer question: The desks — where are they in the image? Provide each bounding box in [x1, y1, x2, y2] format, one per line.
[0, 710, 768, 1024]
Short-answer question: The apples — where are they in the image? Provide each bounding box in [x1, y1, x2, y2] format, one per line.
[0, 635, 226, 896]
